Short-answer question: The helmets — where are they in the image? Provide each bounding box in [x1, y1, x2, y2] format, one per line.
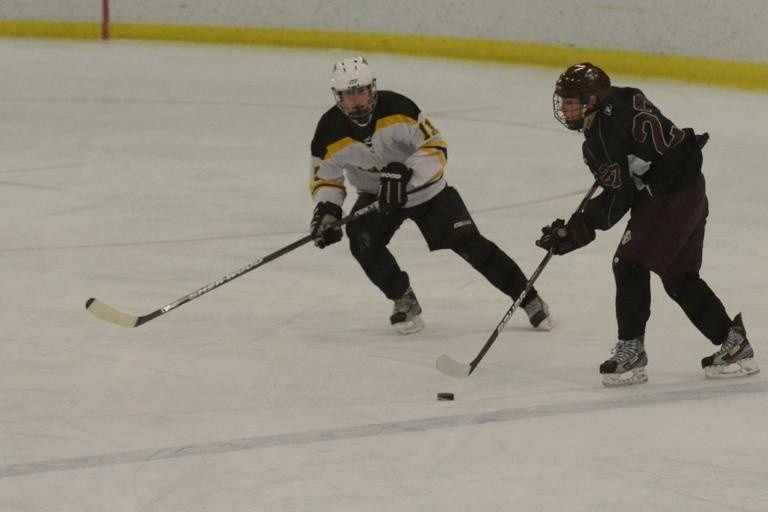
[331, 57, 379, 120]
[552, 63, 609, 130]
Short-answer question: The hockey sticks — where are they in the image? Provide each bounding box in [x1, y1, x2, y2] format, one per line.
[85, 200, 380, 328]
[435, 181, 602, 379]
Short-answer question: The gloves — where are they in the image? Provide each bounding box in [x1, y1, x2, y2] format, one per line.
[535, 214, 593, 255]
[310, 202, 343, 248]
[377, 163, 409, 212]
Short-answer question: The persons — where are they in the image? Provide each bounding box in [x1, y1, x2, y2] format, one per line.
[308, 58, 550, 329]
[534, 61, 755, 374]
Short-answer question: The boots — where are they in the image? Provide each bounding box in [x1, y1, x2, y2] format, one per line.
[389, 284, 421, 324]
[599, 332, 648, 375]
[702, 312, 753, 368]
[519, 290, 549, 327]
